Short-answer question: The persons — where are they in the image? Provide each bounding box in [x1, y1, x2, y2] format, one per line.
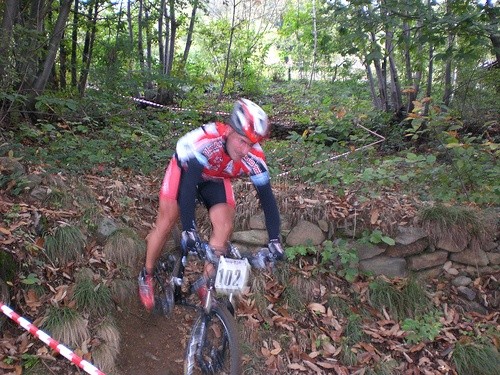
[137, 98, 285, 311]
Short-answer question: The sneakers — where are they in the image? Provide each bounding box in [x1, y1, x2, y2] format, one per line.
[139, 273, 154, 309]
[198, 285, 215, 306]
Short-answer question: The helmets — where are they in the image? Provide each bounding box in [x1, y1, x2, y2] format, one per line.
[230, 97, 268, 143]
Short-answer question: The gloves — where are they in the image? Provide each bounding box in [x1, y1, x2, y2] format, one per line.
[183, 232, 201, 255]
[270, 239, 285, 259]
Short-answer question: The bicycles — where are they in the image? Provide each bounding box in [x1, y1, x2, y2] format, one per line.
[141, 185, 290, 375]
